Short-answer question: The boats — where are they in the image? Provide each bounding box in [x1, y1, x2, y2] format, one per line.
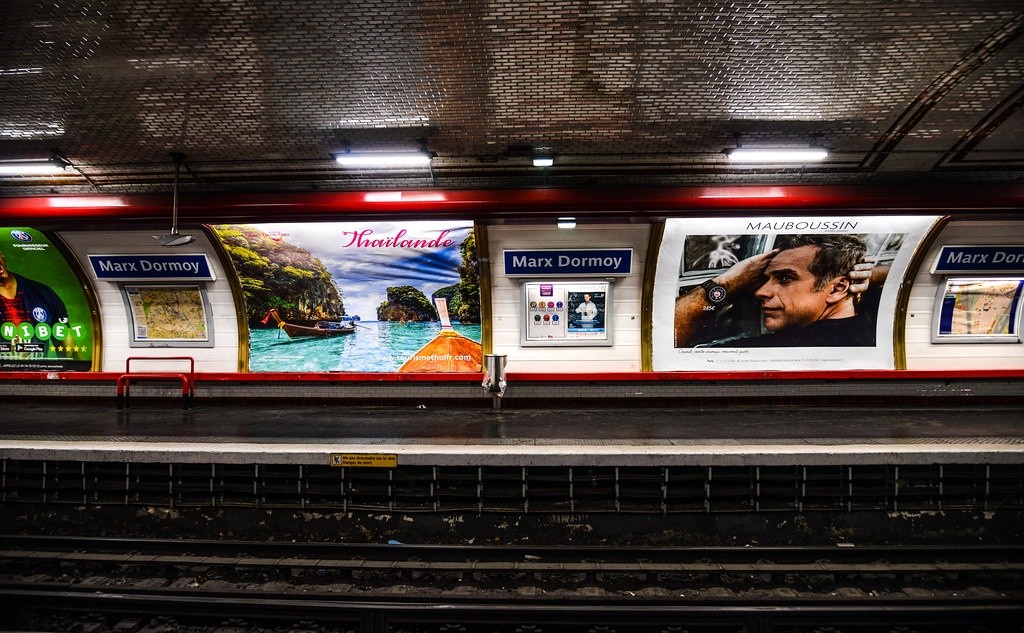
[265, 305, 360, 338]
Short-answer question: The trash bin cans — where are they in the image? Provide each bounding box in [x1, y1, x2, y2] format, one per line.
[483, 355, 507, 393]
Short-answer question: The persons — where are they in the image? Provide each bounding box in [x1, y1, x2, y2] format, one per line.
[576, 294, 598, 328]
[0, 248, 76, 359]
[674, 234, 891, 348]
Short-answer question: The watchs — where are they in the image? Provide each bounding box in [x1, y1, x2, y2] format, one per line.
[700, 279, 728, 311]
